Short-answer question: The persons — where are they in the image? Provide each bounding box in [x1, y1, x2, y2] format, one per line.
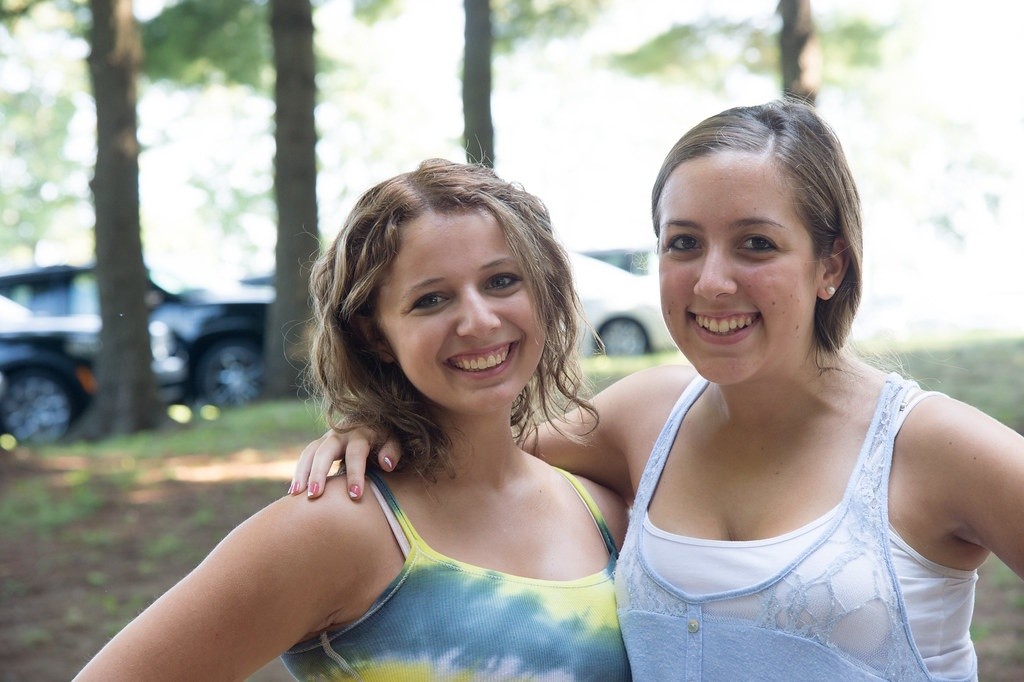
[70, 161, 634, 681]
[289, 98, 1023, 681]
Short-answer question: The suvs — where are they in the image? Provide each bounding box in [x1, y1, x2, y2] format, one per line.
[0, 262, 276, 409]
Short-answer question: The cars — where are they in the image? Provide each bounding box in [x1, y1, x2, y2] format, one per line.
[0, 292, 189, 447]
[556, 248, 681, 360]
